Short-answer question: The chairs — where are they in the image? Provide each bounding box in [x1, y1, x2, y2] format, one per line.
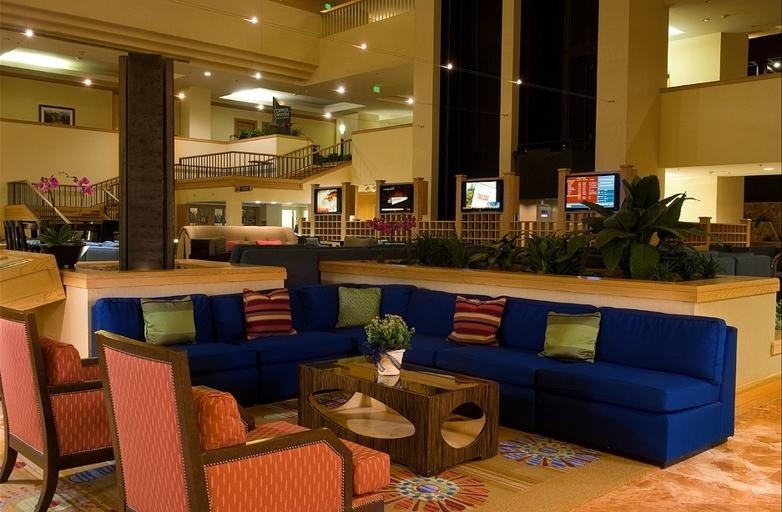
[0, 220, 41, 252]
[700, 251, 782, 277]
[243, 327, 244, 331]
[0, 305, 114, 512]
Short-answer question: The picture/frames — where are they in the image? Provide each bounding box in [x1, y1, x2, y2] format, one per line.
[39, 104, 75, 127]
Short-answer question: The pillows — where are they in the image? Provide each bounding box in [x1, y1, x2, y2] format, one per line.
[289, 286, 339, 331]
[537, 312, 601, 364]
[335, 287, 381, 328]
[445, 296, 505, 348]
[140, 299, 195, 345]
[242, 288, 297, 340]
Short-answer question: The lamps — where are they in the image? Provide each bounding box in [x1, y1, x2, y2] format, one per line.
[256, 105, 264, 110]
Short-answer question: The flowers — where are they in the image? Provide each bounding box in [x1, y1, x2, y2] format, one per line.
[363, 314, 415, 364]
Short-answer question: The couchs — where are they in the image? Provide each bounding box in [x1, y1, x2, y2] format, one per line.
[92, 284, 738, 468]
[93, 331, 389, 512]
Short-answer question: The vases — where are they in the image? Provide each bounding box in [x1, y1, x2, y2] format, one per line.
[377, 349, 405, 375]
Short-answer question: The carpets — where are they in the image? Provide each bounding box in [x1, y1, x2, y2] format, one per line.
[0, 395, 662, 512]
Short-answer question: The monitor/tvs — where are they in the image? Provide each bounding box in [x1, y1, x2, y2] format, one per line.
[564, 173, 620, 212]
[378, 183, 414, 214]
[314, 186, 340, 215]
[460, 178, 503, 212]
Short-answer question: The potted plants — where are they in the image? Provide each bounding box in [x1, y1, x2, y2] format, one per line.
[215, 213, 222, 226]
[239, 130, 247, 139]
[252, 128, 263, 137]
[582, 173, 724, 277]
[188, 212, 200, 226]
[38, 225, 85, 271]
